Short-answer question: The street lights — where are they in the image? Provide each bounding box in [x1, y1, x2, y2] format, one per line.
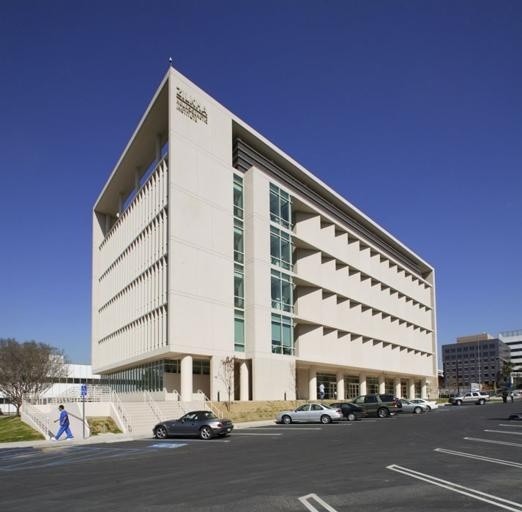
[477, 331, 490, 384]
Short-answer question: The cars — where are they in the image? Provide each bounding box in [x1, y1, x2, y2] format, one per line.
[153, 410, 234, 439]
[272, 392, 439, 423]
[511, 388, 522, 397]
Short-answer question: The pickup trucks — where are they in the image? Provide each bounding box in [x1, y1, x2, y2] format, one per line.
[448, 391, 489, 404]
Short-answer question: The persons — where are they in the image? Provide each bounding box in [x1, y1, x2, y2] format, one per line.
[51, 404, 75, 441]
[509, 391, 515, 403]
